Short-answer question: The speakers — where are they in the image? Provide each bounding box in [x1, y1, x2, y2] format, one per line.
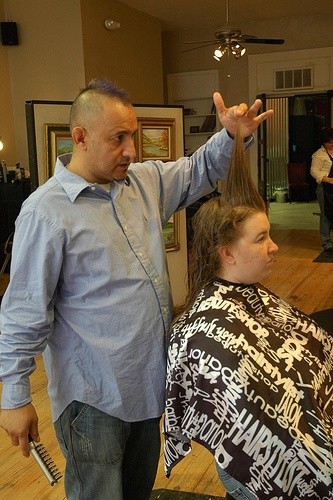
[0, 21, 19, 46]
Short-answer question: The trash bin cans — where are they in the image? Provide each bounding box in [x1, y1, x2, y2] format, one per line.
[275, 188, 287, 203]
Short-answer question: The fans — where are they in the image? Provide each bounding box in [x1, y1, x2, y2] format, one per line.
[181, 0, 285, 53]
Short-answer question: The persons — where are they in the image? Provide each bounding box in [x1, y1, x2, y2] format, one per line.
[0, 80, 274, 500]
[310, 128, 333, 252]
[161, 134, 333, 500]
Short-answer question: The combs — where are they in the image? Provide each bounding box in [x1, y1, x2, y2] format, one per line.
[28, 433, 62, 486]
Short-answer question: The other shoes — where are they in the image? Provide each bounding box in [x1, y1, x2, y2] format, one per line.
[324, 247, 333, 255]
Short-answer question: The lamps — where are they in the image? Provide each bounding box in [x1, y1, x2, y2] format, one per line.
[213, 42, 246, 62]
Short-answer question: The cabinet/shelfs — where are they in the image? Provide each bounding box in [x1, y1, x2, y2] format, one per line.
[175, 94, 217, 156]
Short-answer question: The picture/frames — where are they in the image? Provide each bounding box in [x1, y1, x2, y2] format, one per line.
[44, 116, 180, 253]
